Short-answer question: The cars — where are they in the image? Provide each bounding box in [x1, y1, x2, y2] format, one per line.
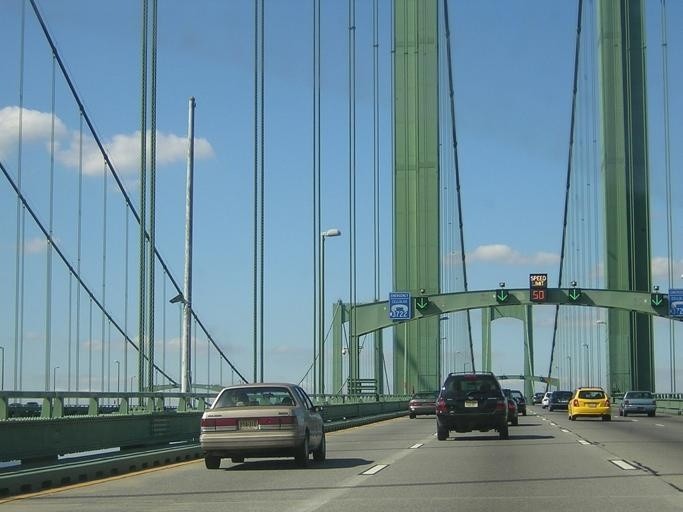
[567, 386, 611, 421]
[435, 371, 526, 441]
[619, 391, 656, 417]
[408, 392, 439, 419]
[199, 383, 326, 469]
[533, 391, 573, 412]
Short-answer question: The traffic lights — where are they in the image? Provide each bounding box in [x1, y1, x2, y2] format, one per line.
[496, 289, 509, 303]
[530, 273, 548, 302]
[651, 293, 664, 307]
[416, 296, 429, 310]
[569, 289, 581, 303]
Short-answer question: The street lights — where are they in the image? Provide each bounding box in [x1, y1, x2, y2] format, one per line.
[567, 355, 571, 391]
[597, 320, 609, 393]
[582, 343, 590, 387]
[318, 230, 341, 392]
